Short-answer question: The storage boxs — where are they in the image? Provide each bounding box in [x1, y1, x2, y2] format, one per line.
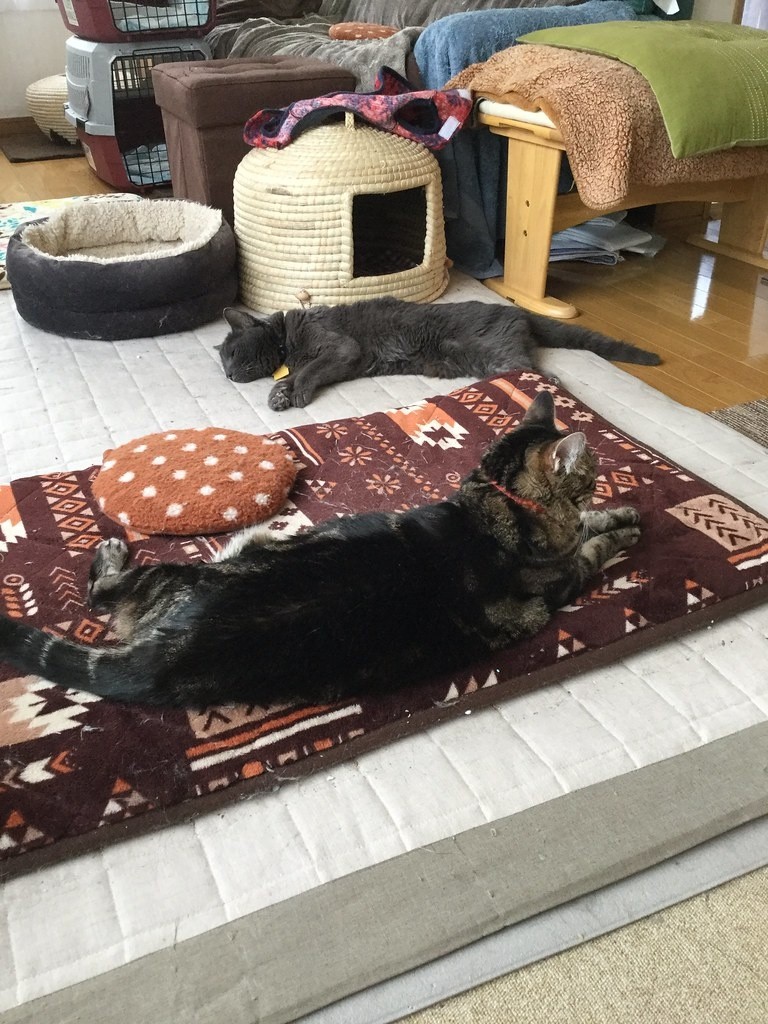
[63, 34, 218, 195]
[55, 0, 217, 43]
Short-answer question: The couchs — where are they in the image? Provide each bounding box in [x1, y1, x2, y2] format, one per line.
[203, 1, 664, 279]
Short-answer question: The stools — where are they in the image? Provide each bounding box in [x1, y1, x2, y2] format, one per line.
[152, 55, 355, 235]
[470, 20, 768, 320]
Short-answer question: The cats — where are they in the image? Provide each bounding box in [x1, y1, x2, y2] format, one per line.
[213, 294, 662, 412]
[1, 390, 646, 706]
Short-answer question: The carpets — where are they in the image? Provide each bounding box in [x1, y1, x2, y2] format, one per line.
[0, 134, 86, 163]
[0, 371, 767, 887]
